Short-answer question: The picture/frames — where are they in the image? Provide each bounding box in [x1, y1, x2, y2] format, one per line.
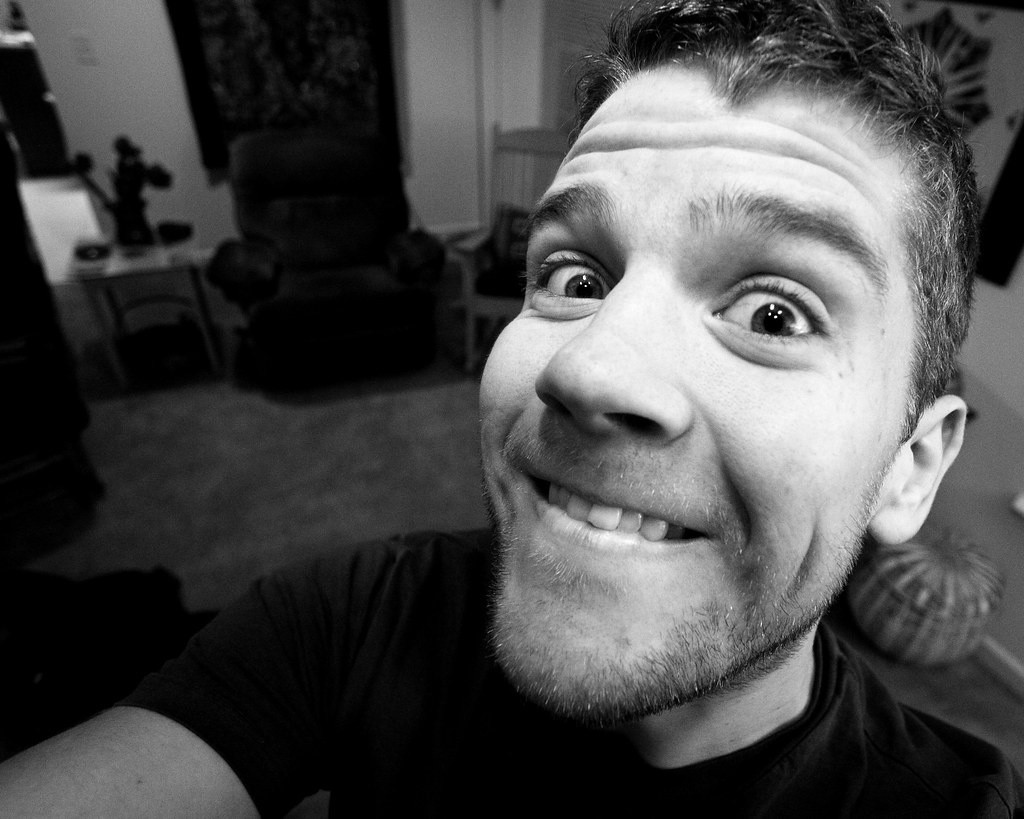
[877, 0, 1024, 290]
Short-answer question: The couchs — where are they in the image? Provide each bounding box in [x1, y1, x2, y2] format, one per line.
[207, 131, 446, 395]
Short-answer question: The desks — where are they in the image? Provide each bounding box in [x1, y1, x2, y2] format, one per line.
[65, 238, 227, 396]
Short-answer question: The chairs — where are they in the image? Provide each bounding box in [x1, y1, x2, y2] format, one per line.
[443, 124, 567, 371]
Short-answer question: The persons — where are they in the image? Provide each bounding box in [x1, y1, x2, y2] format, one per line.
[0, 1, 1024, 819]
